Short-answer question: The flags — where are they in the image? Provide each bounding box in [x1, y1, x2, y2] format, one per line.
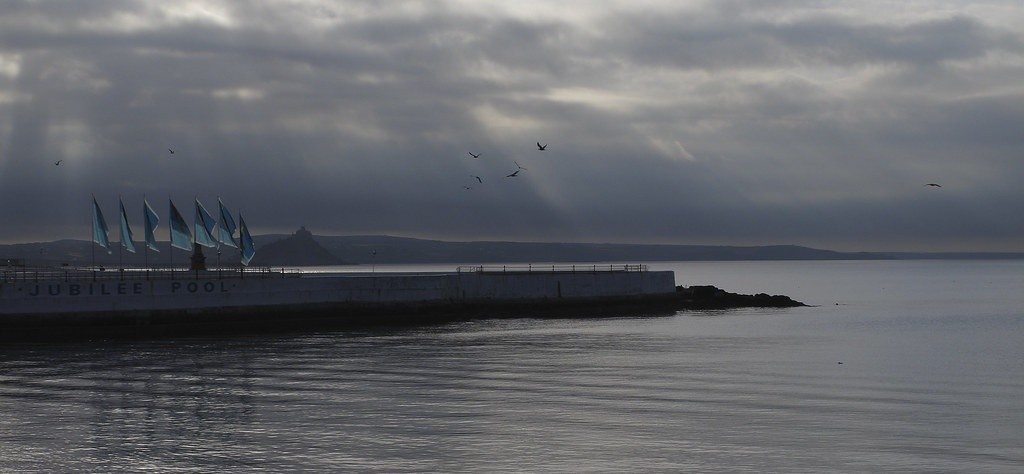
[143, 199, 159, 251]
[195, 200, 219, 248]
[169, 200, 192, 251]
[93, 195, 110, 249]
[238, 215, 255, 265]
[119, 199, 136, 253]
[218, 199, 238, 248]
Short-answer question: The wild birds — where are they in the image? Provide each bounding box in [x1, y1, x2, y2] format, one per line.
[56, 159, 62, 166]
[536, 142, 547, 151]
[462, 150, 483, 191]
[506, 160, 527, 178]
[923, 183, 943, 188]
[169, 149, 174, 154]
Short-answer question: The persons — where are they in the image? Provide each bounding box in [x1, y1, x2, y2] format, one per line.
[100, 265, 105, 271]
